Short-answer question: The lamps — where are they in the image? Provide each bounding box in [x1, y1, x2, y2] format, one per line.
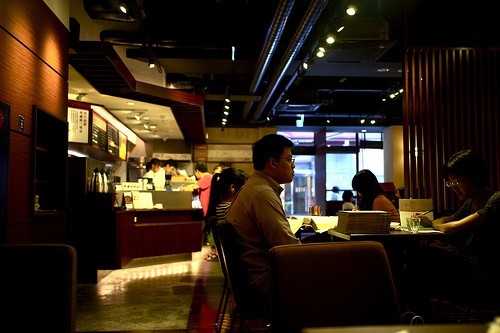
[294, 70, 306, 85]
[280, 89, 294, 103]
[302, 53, 314, 69]
[266, 114, 273, 121]
[331, 14, 344, 33]
[221, 87, 232, 126]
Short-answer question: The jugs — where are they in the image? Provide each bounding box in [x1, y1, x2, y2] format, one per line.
[90, 168, 109, 193]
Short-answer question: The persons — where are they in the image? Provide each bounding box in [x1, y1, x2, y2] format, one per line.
[212, 164, 225, 175]
[342, 190, 356, 211]
[395, 186, 406, 200]
[351, 169, 400, 222]
[202, 167, 251, 248]
[411, 149, 500, 324]
[179, 159, 219, 261]
[142, 157, 162, 183]
[332, 186, 342, 201]
[226, 134, 302, 333]
[152, 158, 177, 192]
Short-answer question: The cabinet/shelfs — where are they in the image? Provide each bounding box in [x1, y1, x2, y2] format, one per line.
[32, 104, 70, 214]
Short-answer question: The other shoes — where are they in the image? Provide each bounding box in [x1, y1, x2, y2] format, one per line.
[410, 315, 424, 325]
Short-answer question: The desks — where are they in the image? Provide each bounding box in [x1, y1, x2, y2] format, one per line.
[301, 323, 500, 333]
[327, 223, 462, 325]
[286, 214, 339, 242]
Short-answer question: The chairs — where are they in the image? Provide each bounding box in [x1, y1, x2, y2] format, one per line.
[209, 215, 301, 333]
[0, 243, 77, 333]
[398, 199, 434, 227]
[325, 200, 355, 216]
[268, 240, 404, 333]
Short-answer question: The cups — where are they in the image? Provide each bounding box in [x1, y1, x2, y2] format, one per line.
[406, 218, 421, 231]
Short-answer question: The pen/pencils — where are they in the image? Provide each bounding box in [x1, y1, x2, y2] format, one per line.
[419, 208, 435, 216]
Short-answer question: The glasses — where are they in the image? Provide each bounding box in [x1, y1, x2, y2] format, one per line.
[446, 176, 463, 187]
[278, 157, 295, 163]
